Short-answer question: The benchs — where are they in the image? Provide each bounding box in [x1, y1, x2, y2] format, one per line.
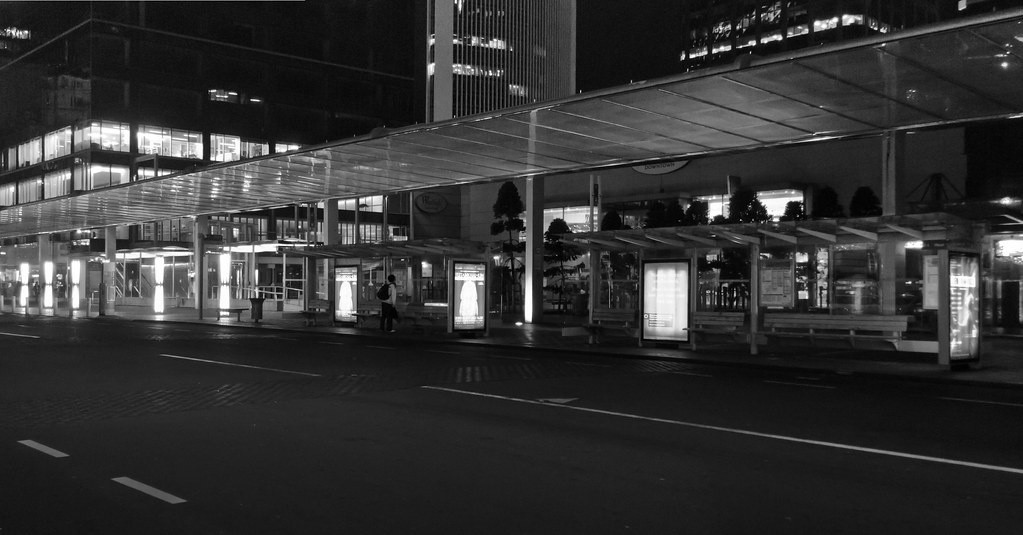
[687, 310, 746, 352]
[299, 300, 333, 327]
[583, 307, 638, 346]
[352, 302, 448, 329]
[215, 308, 249, 322]
[753, 311, 914, 353]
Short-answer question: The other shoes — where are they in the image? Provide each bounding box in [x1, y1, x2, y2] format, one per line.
[388, 330, 396, 332]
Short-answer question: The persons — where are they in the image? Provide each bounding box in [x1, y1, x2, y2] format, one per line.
[379, 274, 396, 332]
[34, 282, 39, 302]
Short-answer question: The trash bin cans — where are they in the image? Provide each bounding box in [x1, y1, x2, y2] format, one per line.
[248, 297, 265, 319]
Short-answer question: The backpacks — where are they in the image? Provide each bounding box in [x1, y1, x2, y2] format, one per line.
[377, 281, 396, 300]
